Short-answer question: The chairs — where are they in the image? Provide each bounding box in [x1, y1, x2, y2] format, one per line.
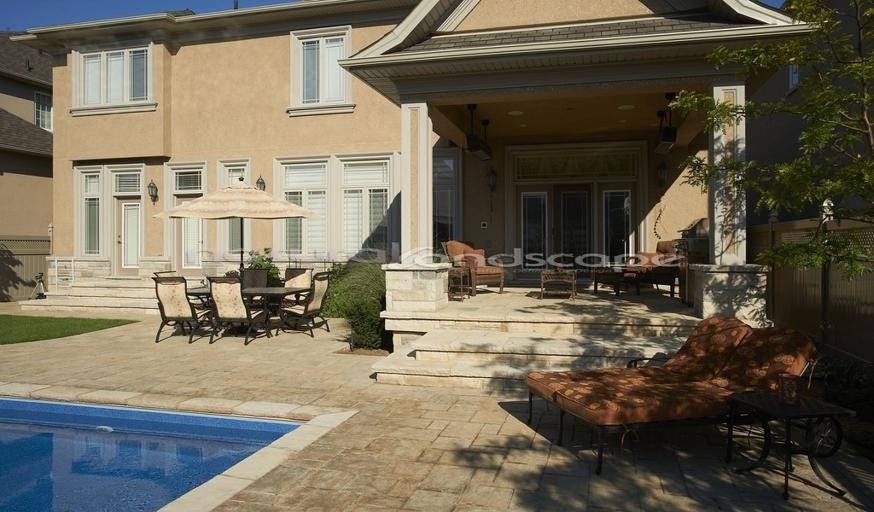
[150, 266, 330, 346]
[440, 238, 504, 297]
[540, 268, 578, 300]
[624, 240, 677, 297]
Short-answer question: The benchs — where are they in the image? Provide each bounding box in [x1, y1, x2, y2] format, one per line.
[593, 266, 641, 298]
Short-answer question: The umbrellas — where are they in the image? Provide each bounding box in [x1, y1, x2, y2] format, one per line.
[150, 175, 316, 288]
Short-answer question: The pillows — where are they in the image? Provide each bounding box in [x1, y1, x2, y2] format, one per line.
[462, 248, 485, 269]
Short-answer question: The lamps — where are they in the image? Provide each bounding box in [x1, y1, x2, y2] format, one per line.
[254, 175, 265, 191]
[652, 93, 677, 155]
[465, 104, 493, 162]
[655, 157, 669, 188]
[146, 179, 158, 206]
[487, 168, 497, 191]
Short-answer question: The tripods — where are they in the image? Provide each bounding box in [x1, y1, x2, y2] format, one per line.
[29, 278, 48, 299]
